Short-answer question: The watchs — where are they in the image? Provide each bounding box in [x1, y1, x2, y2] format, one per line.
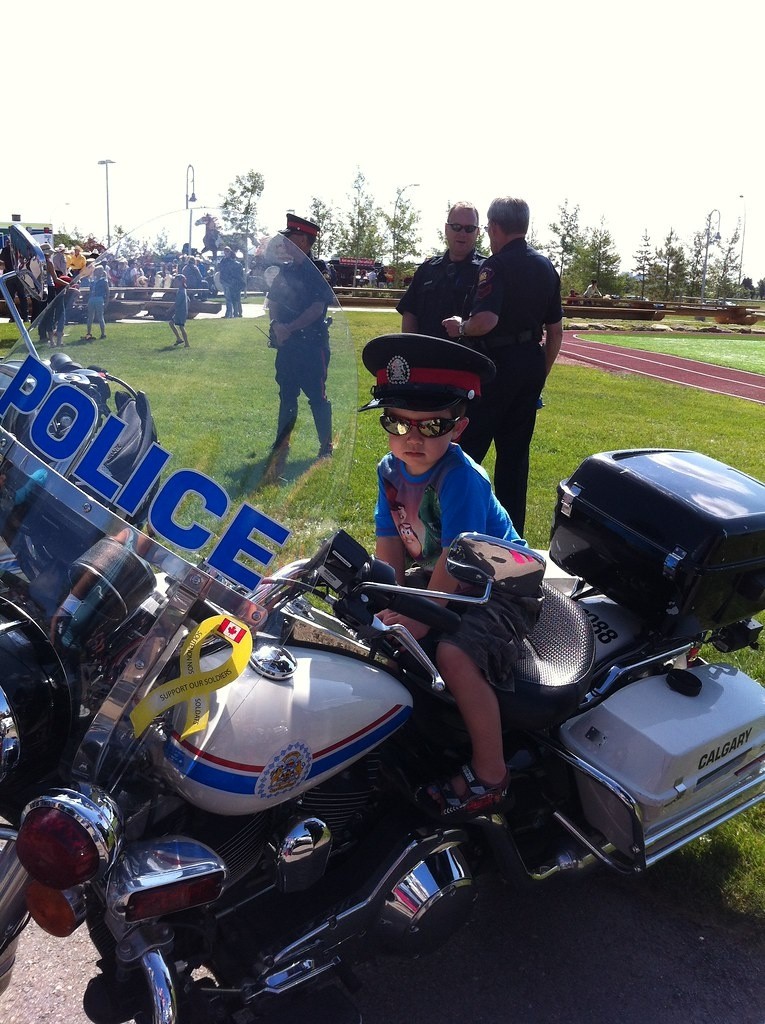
[458, 322, 467, 335]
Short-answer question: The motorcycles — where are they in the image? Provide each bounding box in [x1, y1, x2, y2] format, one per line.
[0, 208, 765, 1023]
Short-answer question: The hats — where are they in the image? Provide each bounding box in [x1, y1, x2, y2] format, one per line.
[265, 235, 288, 267]
[357, 333, 497, 411]
[278, 213, 321, 236]
[73, 245, 83, 252]
[176, 274, 188, 288]
[40, 243, 56, 253]
[55, 243, 67, 250]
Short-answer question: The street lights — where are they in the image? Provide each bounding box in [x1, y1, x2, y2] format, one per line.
[393, 183, 420, 287]
[185, 162, 199, 208]
[700, 209, 721, 309]
[97, 160, 116, 247]
[738, 195, 747, 287]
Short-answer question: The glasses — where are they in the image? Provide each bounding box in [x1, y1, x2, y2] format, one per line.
[484, 219, 496, 232]
[380, 412, 464, 438]
[447, 223, 479, 232]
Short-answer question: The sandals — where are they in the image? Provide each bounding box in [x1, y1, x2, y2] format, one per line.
[174, 340, 184, 345]
[414, 759, 511, 821]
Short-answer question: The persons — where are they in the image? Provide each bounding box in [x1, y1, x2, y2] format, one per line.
[567, 289, 580, 304]
[427, 419, 449, 436]
[441, 196, 563, 541]
[53, 244, 209, 299]
[398, 422, 406, 434]
[356, 267, 387, 287]
[220, 246, 243, 318]
[357, 333, 545, 820]
[167, 275, 190, 347]
[86, 265, 110, 339]
[0, 237, 79, 348]
[267, 212, 332, 453]
[582, 280, 602, 305]
[395, 200, 489, 343]
[326, 263, 337, 286]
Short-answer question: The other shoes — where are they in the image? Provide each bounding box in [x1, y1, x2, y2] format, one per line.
[48, 340, 65, 348]
[269, 442, 287, 451]
[35, 339, 48, 343]
[223, 316, 233, 319]
[234, 313, 243, 317]
[318, 447, 333, 458]
